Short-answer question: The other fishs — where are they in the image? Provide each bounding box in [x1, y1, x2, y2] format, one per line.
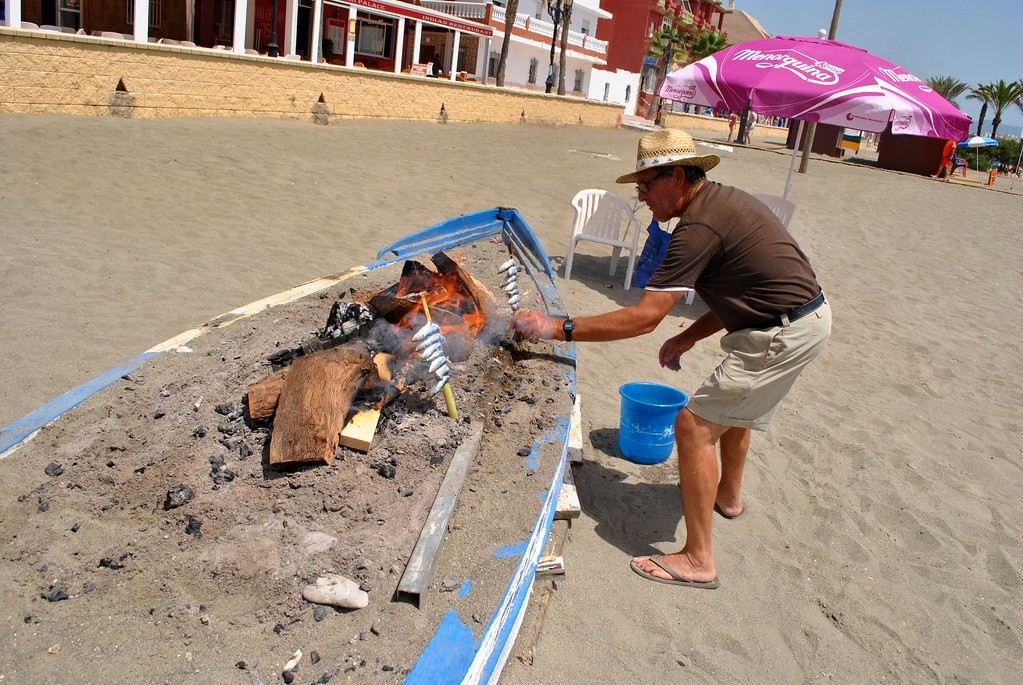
[496, 259, 521, 310]
[411, 322, 455, 400]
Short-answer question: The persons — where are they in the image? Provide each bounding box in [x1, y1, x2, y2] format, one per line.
[727, 111, 740, 143]
[511, 129, 833, 589]
[743, 107, 757, 144]
[930, 140, 956, 182]
[988, 158, 1022, 187]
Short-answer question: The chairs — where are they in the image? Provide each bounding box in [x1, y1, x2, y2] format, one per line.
[354, 62, 364, 67]
[402, 69, 477, 82]
[950, 153, 968, 177]
[148, 36, 234, 52]
[21, 20, 134, 41]
[243, 48, 302, 62]
[752, 193, 796, 231]
[332, 60, 345, 65]
[564, 188, 641, 290]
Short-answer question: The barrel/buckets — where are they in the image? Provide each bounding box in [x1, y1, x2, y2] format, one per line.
[618, 382, 688, 465]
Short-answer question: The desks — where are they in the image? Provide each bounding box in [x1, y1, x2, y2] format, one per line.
[623, 196, 670, 242]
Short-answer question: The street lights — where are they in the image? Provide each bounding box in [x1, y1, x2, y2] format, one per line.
[544, 0, 573, 93]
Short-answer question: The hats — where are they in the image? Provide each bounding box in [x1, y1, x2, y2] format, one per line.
[616, 128, 720, 184]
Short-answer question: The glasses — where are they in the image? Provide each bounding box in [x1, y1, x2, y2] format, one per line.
[635, 166, 674, 193]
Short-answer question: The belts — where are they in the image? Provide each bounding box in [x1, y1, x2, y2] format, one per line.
[753, 290, 824, 330]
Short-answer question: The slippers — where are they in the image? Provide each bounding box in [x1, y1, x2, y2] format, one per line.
[631, 554, 721, 589]
[678, 481, 745, 519]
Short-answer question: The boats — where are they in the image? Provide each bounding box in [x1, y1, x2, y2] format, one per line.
[0, 205, 588, 685]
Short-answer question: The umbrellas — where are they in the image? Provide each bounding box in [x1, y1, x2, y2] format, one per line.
[956, 135, 999, 180]
[654, 28, 973, 198]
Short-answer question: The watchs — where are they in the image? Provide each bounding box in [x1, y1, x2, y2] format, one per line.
[563, 318, 576, 342]
